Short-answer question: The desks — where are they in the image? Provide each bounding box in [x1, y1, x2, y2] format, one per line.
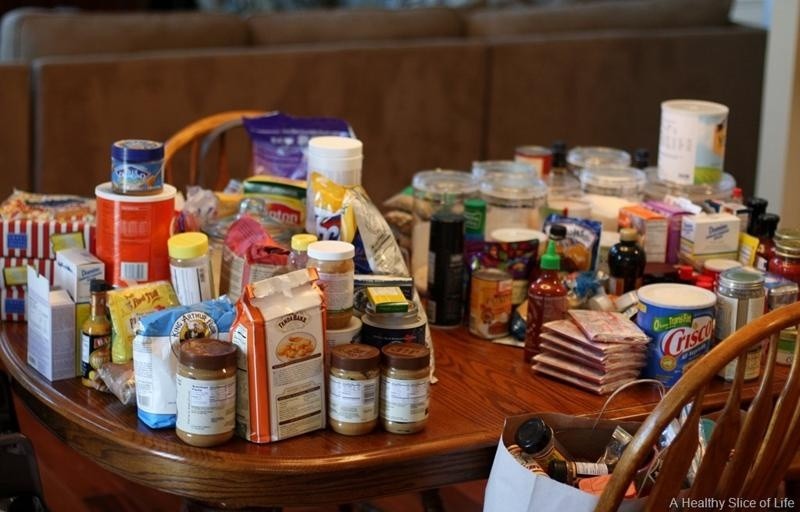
[1, 299, 799, 511]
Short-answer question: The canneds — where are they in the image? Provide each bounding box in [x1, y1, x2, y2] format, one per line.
[379, 343, 429, 434]
[176, 338, 237, 447]
[716, 269, 766, 344]
[466, 267, 510, 338]
[328, 344, 381, 436]
[306, 242, 356, 329]
[512, 143, 554, 182]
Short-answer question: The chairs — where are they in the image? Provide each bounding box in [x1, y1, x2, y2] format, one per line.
[595, 300, 798, 512]
[159, 110, 270, 193]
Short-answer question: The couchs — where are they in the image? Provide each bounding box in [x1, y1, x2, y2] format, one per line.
[0, 1, 768, 198]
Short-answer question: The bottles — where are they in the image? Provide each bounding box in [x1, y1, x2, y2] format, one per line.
[165, 231, 358, 330]
[675, 198, 798, 384]
[516, 416, 616, 482]
[80, 278, 112, 387]
[409, 142, 649, 373]
